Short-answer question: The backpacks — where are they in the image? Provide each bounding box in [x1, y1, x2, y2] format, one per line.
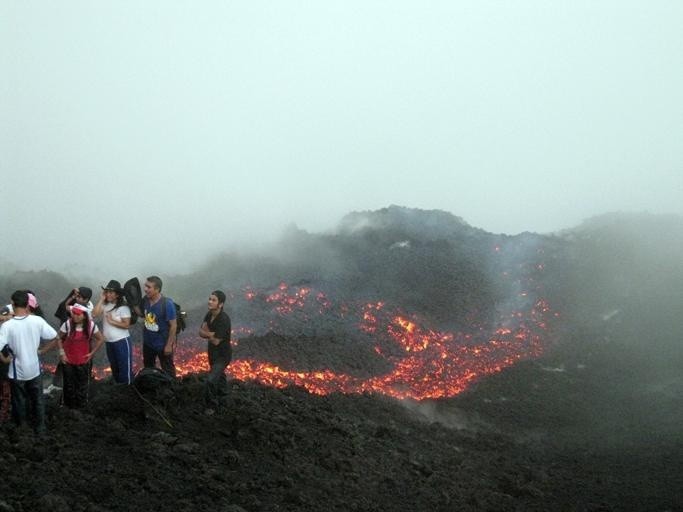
[115, 301, 138, 326]
[140, 293, 188, 334]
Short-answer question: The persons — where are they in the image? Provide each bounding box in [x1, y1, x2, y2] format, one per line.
[53, 285, 96, 386]
[54, 302, 105, 413]
[122, 275, 179, 381]
[92, 280, 133, 388]
[0, 286, 60, 435]
[198, 289, 233, 398]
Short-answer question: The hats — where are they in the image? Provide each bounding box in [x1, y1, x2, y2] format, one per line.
[100, 278, 129, 297]
[25, 292, 37, 309]
[65, 301, 89, 315]
[123, 276, 143, 306]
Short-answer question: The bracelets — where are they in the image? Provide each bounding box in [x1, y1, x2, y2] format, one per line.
[57, 348, 65, 357]
